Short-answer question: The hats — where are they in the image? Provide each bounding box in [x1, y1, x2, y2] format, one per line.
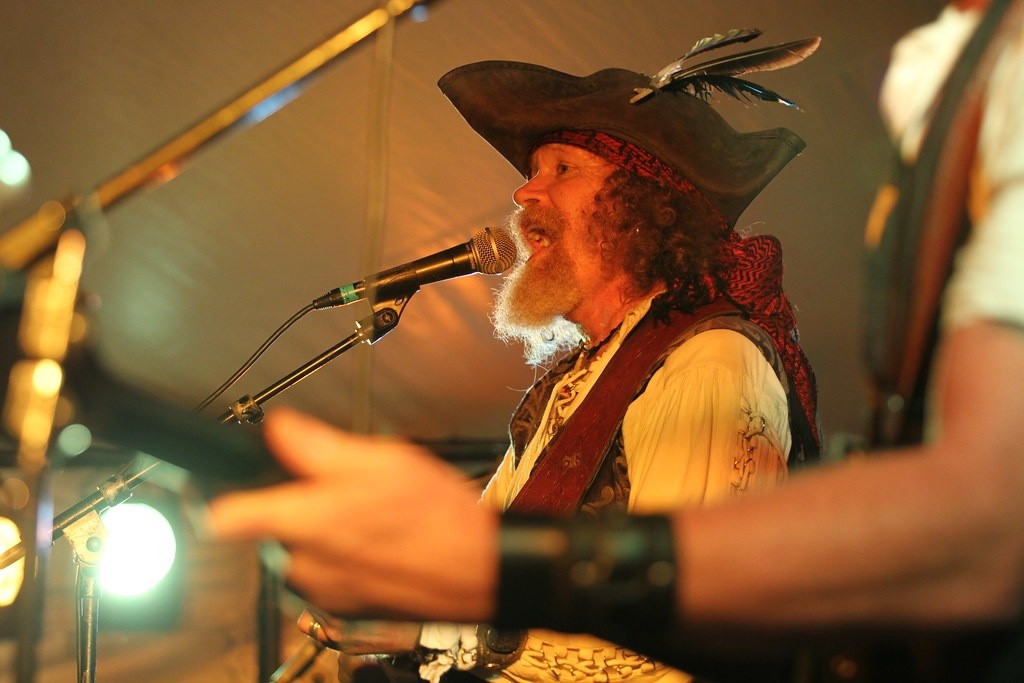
[438, 28, 821, 231]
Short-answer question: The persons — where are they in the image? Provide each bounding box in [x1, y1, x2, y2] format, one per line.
[190, 1, 1024, 683]
[339, 28, 820, 683]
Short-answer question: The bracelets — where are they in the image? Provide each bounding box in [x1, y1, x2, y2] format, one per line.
[498, 507, 676, 652]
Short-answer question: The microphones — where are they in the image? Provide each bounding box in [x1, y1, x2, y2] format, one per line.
[314, 227, 517, 311]
